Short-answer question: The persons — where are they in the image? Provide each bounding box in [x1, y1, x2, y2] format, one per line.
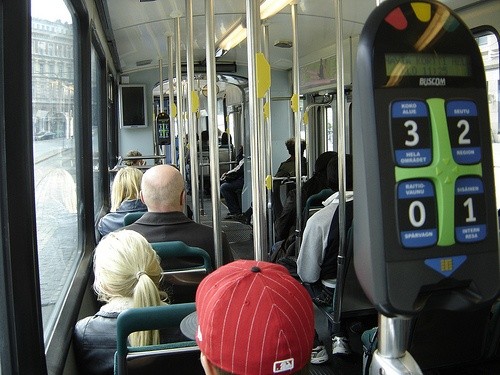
[74, 230, 196, 375]
[33, 166, 79, 261]
[180, 261, 315, 375]
[93, 127, 354, 309]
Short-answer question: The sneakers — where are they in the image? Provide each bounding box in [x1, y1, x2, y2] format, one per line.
[310, 346, 328, 364]
[331, 336, 352, 357]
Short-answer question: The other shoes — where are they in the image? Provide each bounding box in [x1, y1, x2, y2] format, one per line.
[227, 213, 232, 215]
[232, 214, 251, 224]
[223, 217, 237, 221]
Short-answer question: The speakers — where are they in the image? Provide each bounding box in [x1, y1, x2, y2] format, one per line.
[119, 84, 147, 128]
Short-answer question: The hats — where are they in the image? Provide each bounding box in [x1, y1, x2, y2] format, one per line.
[180, 259, 314, 375]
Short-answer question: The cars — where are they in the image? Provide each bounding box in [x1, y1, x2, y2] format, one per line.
[33, 130, 56, 141]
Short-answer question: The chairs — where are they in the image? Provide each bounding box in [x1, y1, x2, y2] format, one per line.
[113, 144, 352, 375]
